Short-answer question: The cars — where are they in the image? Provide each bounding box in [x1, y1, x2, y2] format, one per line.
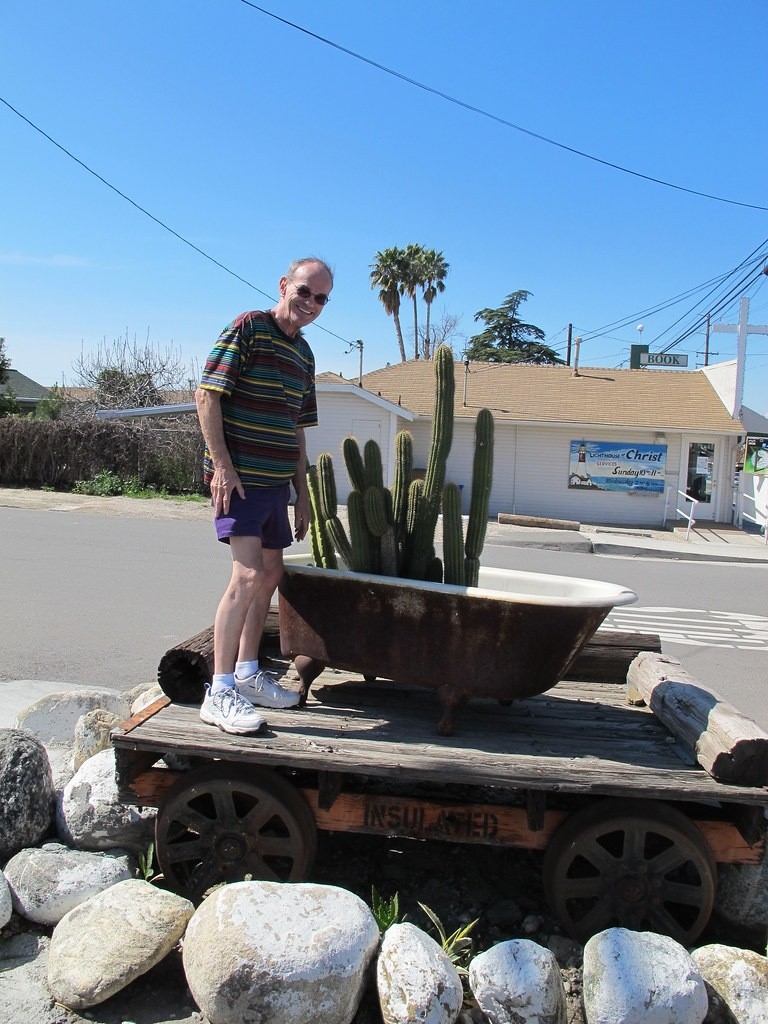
[687, 443, 739, 505]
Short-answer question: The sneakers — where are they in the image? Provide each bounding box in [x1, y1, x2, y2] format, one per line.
[200, 683, 269, 735]
[234, 671, 301, 709]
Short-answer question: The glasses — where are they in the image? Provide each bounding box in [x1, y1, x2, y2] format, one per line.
[288, 276, 331, 306]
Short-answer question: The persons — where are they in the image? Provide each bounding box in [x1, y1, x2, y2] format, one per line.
[195, 259, 335, 733]
